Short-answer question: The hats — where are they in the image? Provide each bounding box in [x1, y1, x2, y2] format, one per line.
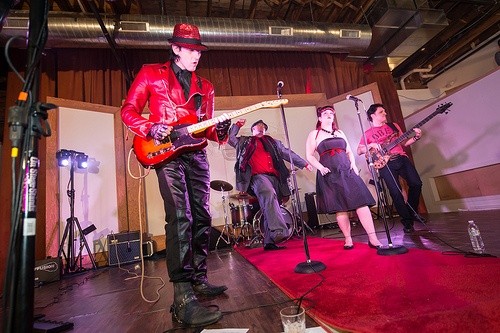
[168, 23, 208, 50]
[251, 120, 268, 132]
[317, 105, 336, 117]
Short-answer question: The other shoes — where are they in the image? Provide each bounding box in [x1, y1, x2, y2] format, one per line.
[264, 243, 278, 251]
[413, 214, 427, 223]
[403, 227, 414, 233]
[273, 232, 287, 244]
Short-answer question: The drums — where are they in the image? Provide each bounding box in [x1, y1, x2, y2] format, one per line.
[229, 203, 254, 229]
[252, 206, 295, 245]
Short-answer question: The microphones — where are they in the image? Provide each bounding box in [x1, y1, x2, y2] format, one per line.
[346, 95, 363, 102]
[277, 80, 284, 90]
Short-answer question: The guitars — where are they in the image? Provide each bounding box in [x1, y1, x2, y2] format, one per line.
[132, 99, 288, 170]
[364, 101, 453, 169]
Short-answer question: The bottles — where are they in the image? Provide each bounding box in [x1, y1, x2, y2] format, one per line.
[468, 220, 485, 251]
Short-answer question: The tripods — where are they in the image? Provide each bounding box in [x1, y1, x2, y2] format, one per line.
[215, 187, 256, 250]
[57, 156, 98, 274]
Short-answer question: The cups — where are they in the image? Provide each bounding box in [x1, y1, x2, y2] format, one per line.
[280, 306, 306, 333]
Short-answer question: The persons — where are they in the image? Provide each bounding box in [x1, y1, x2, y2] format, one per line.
[357, 103, 427, 234]
[305, 105, 388, 249]
[122, 23, 231, 327]
[230, 118, 312, 252]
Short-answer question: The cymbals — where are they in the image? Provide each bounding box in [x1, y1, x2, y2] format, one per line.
[225, 193, 250, 200]
[210, 179, 234, 192]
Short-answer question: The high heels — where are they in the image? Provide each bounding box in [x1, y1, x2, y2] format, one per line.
[368, 241, 384, 249]
[344, 244, 353, 249]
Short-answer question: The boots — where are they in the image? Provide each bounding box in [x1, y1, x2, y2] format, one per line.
[170, 282, 223, 329]
[192, 282, 228, 300]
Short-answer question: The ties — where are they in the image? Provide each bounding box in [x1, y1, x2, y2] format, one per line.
[183, 71, 190, 102]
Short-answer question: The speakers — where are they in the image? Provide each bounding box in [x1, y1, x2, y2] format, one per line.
[107, 231, 142, 266]
[305, 193, 338, 226]
